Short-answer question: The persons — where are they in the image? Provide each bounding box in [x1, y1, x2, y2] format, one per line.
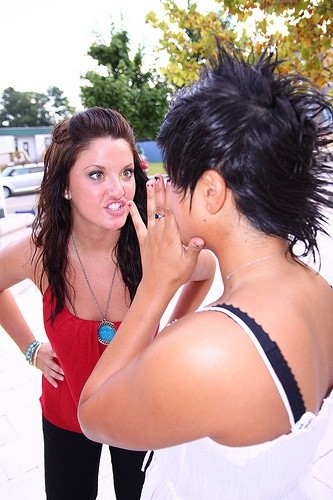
[0, 107, 216, 499]
[78, 34, 333, 500]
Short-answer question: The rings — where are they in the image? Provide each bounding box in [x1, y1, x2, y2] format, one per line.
[154, 213, 165, 220]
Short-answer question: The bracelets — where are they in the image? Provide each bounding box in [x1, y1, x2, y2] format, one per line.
[25, 340, 44, 369]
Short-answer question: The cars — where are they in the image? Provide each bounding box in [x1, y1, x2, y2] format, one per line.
[0, 162, 51, 199]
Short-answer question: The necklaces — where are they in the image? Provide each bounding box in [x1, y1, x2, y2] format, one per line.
[223, 252, 285, 285]
[70, 232, 118, 346]
